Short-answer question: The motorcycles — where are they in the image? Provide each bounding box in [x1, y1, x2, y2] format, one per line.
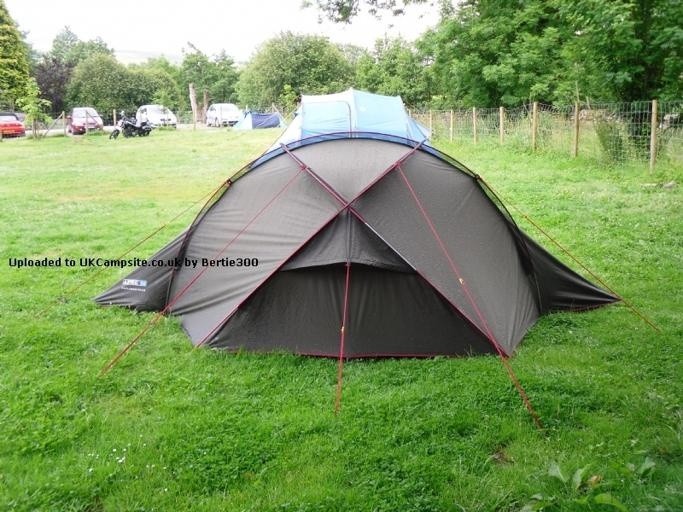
[109, 111, 152, 139]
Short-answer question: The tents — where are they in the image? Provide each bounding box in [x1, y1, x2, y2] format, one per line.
[93, 88, 624, 359]
[231, 108, 281, 132]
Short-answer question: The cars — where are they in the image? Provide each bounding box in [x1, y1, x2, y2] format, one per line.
[0, 112, 25, 139]
[205, 103, 244, 127]
[65, 107, 103, 135]
[136, 104, 177, 129]
